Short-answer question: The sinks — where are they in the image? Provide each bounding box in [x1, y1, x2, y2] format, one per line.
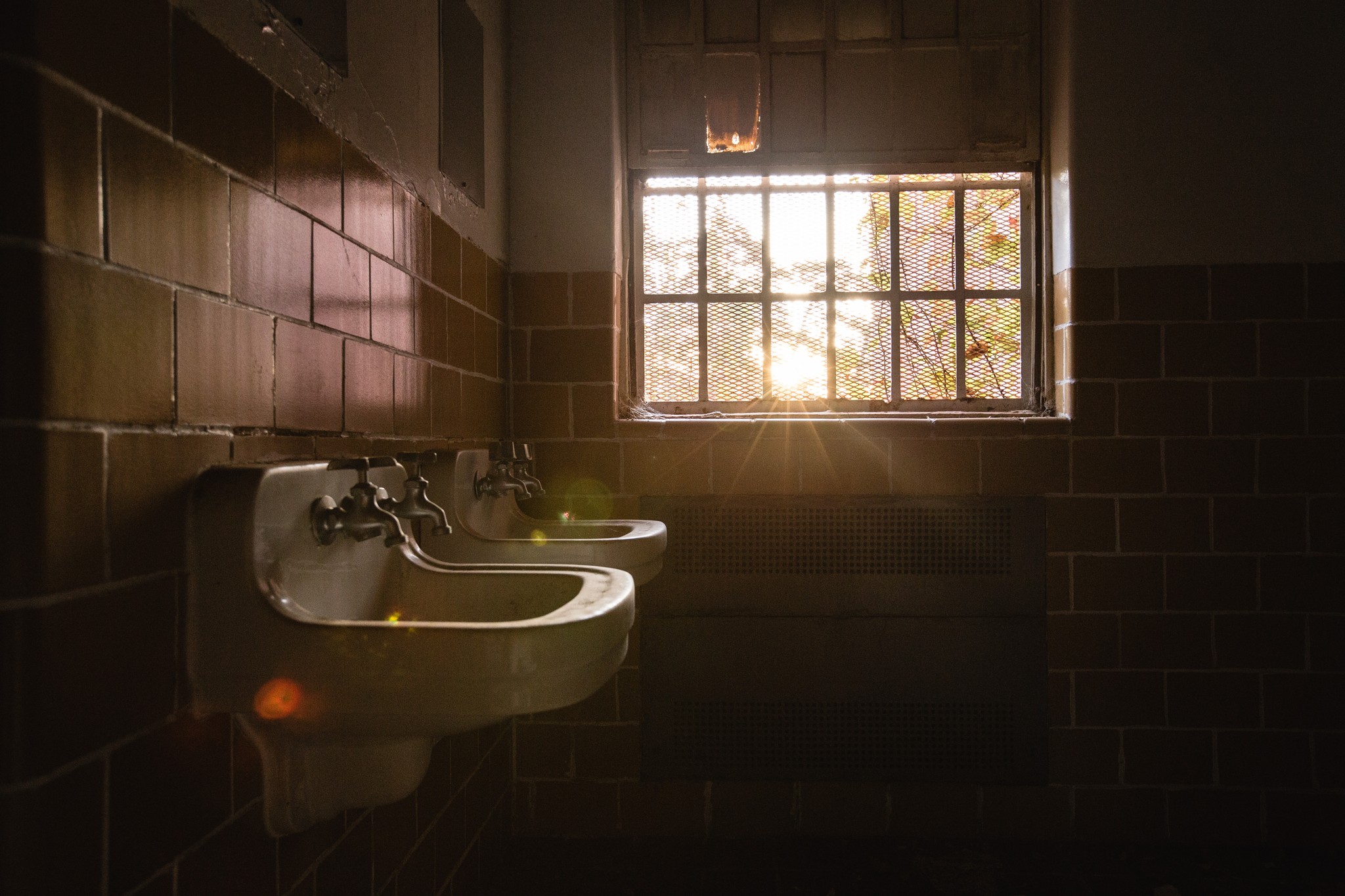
[187, 450, 640, 845]
[411, 442, 670, 594]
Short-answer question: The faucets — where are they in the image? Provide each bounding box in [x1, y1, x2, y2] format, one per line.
[472, 442, 532, 503]
[310, 455, 413, 552]
[372, 451, 455, 537]
[492, 443, 549, 498]
